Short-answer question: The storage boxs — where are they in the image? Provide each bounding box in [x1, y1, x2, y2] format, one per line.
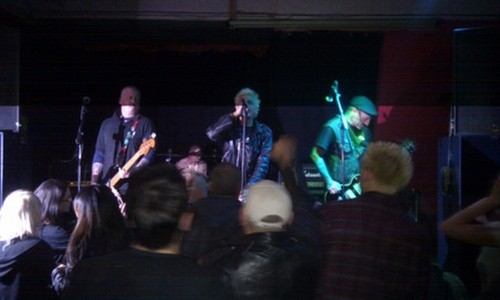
[277, 164, 326, 195]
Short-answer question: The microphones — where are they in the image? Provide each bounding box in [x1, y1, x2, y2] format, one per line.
[326, 80, 339, 102]
[243, 99, 250, 118]
[83, 96, 89, 105]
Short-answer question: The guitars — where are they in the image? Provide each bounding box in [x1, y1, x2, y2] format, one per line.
[323, 139, 417, 202]
[105, 137, 156, 189]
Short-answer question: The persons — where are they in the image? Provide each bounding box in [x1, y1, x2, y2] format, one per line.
[176, 145, 205, 183]
[0, 136, 500, 300]
[311, 95, 379, 200]
[206, 87, 272, 202]
[90, 87, 155, 200]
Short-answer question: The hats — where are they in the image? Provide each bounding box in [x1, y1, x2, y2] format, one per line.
[247, 179, 294, 230]
[350, 96, 378, 118]
[119, 87, 140, 107]
